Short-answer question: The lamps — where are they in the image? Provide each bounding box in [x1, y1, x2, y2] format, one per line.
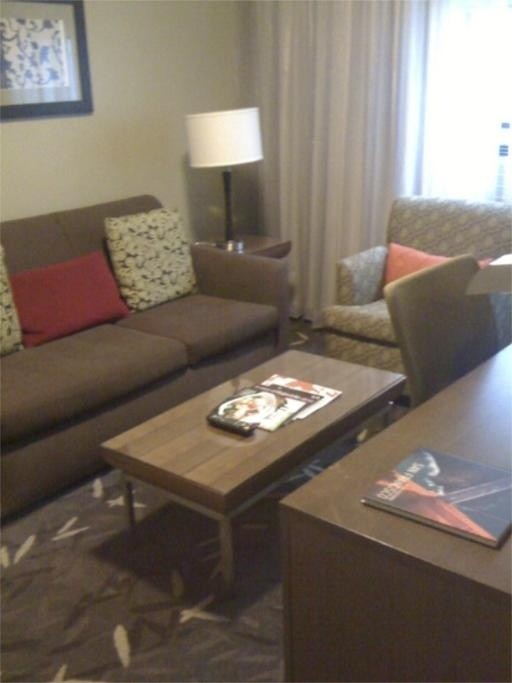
[183, 106, 266, 252]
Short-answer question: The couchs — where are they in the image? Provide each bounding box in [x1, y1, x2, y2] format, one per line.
[0, 194, 294, 520]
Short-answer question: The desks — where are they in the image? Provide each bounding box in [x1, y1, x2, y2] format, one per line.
[100, 344, 410, 590]
[276, 343, 512, 683]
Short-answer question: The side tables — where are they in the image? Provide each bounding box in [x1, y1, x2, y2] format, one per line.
[204, 230, 294, 258]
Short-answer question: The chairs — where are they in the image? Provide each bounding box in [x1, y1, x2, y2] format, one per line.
[321, 196, 512, 399]
[383, 252, 501, 406]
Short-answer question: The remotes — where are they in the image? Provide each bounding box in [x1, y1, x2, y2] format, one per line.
[207, 414, 256, 435]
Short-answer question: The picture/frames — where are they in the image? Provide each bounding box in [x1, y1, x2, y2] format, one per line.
[0, 1, 94, 121]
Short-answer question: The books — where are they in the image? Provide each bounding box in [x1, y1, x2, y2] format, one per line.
[264, 373, 343, 423]
[215, 382, 306, 433]
[361, 443, 511, 549]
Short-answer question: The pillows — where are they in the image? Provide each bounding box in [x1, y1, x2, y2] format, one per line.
[9, 249, 132, 348]
[103, 206, 198, 312]
[386, 240, 493, 283]
[0, 243, 26, 356]
